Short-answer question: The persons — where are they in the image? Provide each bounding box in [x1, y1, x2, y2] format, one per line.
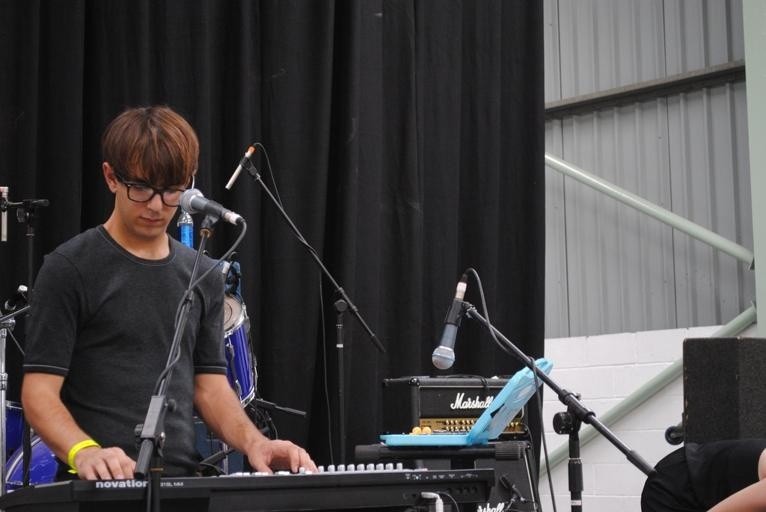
[20, 106, 321, 483]
[641, 438, 766, 512]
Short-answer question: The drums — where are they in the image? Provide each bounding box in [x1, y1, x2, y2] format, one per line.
[192, 291, 262, 423]
[6, 400, 60, 488]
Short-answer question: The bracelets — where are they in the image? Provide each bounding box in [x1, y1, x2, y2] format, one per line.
[66, 439, 100, 475]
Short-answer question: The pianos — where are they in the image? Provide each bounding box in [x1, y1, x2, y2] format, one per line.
[1, 460, 495, 511]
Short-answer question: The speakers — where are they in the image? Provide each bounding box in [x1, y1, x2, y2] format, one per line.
[681, 336, 766, 440]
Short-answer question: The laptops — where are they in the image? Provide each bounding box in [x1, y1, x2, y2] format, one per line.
[379, 356, 553, 448]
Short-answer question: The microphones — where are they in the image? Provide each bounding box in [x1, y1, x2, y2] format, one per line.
[225, 145, 256, 191]
[176, 188, 245, 227]
[0, 186, 10, 242]
[4, 285, 30, 311]
[430, 273, 470, 370]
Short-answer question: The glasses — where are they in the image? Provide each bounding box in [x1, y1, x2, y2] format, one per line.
[117, 173, 196, 207]
